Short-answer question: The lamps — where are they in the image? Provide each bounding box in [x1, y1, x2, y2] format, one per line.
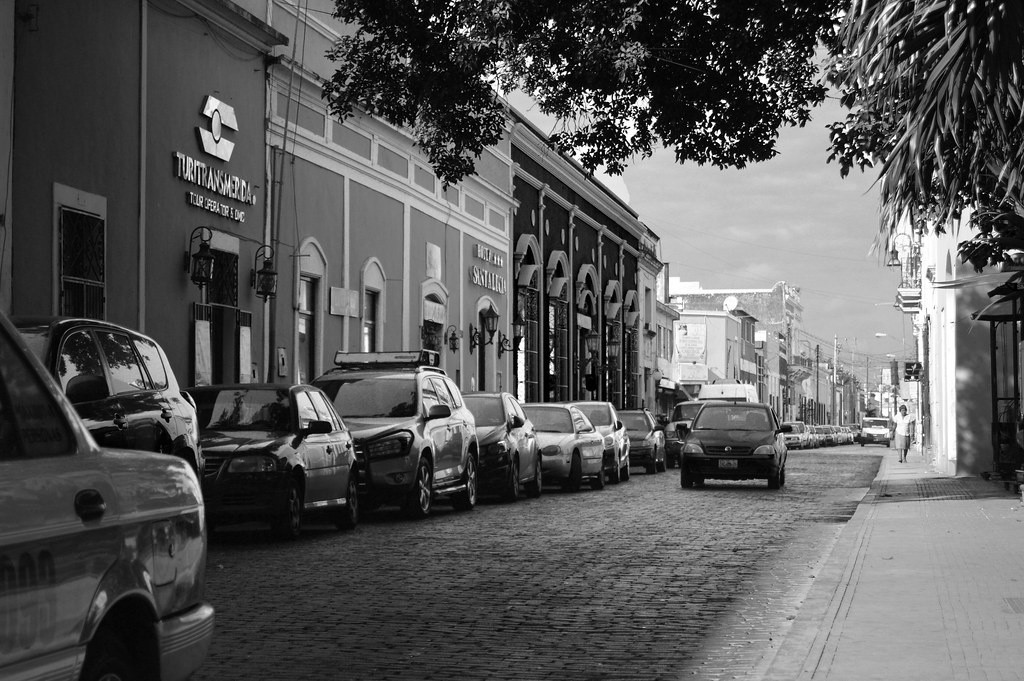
[645, 368, 662, 380]
[183, 225, 216, 290]
[421, 318, 437, 350]
[573, 323, 601, 373]
[468, 300, 500, 354]
[498, 310, 528, 358]
[595, 334, 621, 374]
[444, 324, 458, 354]
[250, 244, 277, 303]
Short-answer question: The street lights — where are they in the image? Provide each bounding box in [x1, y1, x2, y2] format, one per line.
[875, 333, 919, 450]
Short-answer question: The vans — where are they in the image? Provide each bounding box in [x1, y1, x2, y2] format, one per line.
[697, 384, 760, 443]
[8, 316, 205, 474]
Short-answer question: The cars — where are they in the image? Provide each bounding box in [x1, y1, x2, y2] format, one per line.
[180, 383, 363, 534]
[512, 402, 606, 492]
[664, 400, 731, 469]
[308, 350, 480, 520]
[564, 401, 631, 484]
[611, 410, 667, 474]
[814, 425, 854, 449]
[857, 417, 891, 448]
[679, 399, 792, 489]
[1, 307, 215, 680]
[782, 421, 818, 450]
[461, 391, 543, 503]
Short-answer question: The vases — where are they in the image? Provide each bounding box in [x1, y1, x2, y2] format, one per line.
[1016, 469, 1024, 482]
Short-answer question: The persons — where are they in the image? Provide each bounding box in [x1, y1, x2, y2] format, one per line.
[890, 405, 915, 463]
